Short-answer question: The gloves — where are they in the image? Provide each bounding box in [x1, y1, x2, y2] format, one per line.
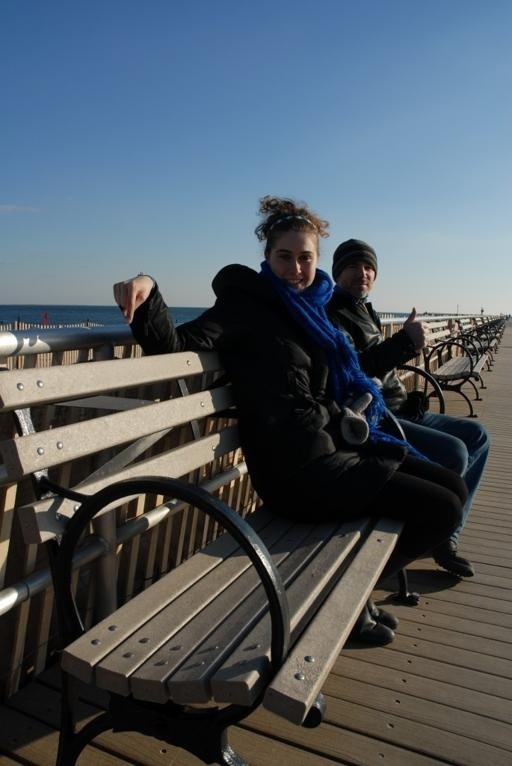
[335, 377, 384, 446]
[394, 389, 431, 424]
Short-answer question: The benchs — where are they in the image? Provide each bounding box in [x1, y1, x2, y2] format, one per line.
[418, 315, 506, 418]
[0, 344, 447, 764]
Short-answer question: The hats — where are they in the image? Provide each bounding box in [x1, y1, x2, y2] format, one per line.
[332, 238, 377, 280]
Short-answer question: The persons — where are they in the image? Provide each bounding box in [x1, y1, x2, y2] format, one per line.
[112, 195, 469, 646]
[329, 239, 490, 578]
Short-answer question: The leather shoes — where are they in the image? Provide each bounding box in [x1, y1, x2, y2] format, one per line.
[434, 537, 475, 575]
[347, 599, 400, 647]
